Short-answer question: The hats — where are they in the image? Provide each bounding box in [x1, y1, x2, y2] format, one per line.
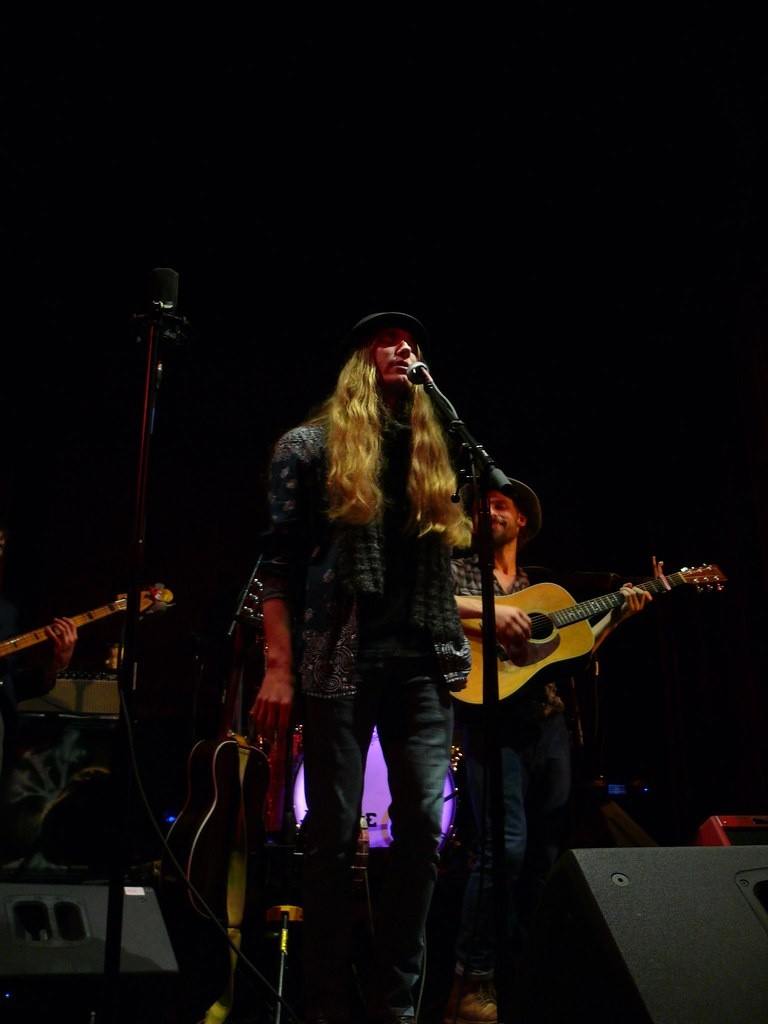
[342, 311, 428, 356]
[458, 476, 542, 547]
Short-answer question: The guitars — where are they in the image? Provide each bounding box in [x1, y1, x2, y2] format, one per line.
[0, 586, 173, 662]
[157, 556, 275, 939]
[449, 562, 728, 721]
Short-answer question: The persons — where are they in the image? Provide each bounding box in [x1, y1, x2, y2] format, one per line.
[196, 312, 653, 1024]
[0, 524, 78, 866]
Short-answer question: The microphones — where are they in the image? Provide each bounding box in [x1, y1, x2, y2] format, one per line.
[144, 267, 180, 391]
[406, 361, 430, 385]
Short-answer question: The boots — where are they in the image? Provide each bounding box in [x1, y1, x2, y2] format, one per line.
[447, 968, 497, 1023]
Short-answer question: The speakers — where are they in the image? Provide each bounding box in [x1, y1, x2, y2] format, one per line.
[0, 882, 179, 1024]
[496, 844, 768, 1024]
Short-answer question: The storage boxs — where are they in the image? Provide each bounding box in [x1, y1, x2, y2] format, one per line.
[47, 678, 121, 715]
